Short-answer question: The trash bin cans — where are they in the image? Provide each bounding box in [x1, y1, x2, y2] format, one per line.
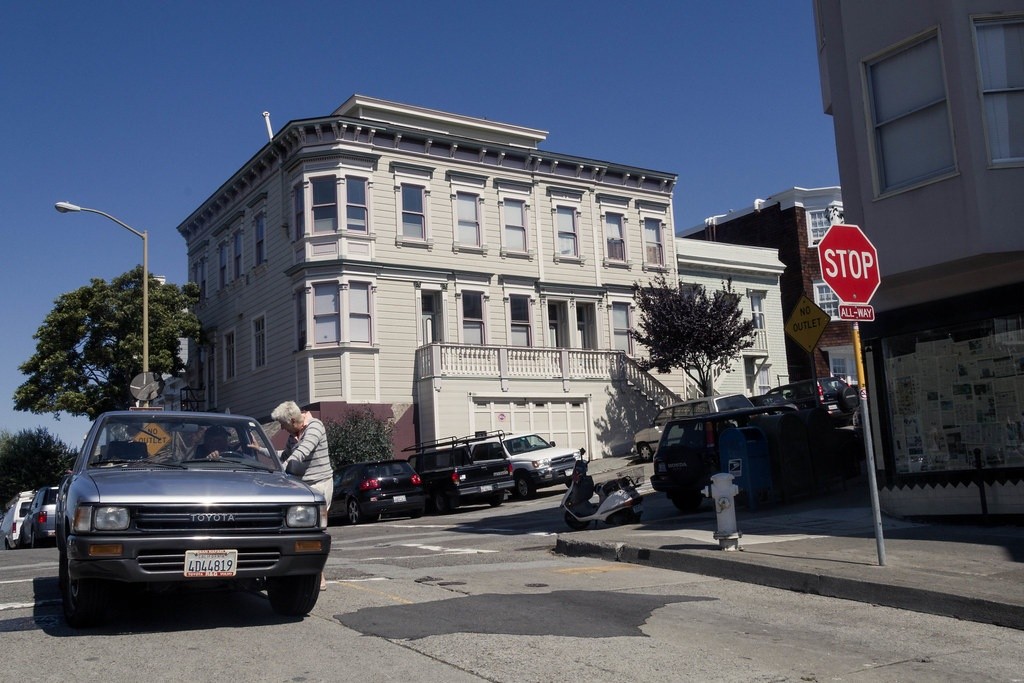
[719, 426, 775, 509]
[752, 407, 849, 505]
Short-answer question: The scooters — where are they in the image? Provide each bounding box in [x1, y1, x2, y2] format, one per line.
[560, 448, 645, 532]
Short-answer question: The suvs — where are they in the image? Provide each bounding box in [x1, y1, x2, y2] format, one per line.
[3, 490, 36, 550]
[55, 409, 332, 619]
[650, 403, 823, 511]
[762, 378, 858, 427]
[633, 393, 756, 464]
[457, 433, 586, 500]
[399, 431, 515, 515]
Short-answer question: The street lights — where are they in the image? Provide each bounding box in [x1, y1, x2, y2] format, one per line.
[55, 200, 150, 409]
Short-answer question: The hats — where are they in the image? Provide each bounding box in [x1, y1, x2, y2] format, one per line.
[203, 426, 232, 439]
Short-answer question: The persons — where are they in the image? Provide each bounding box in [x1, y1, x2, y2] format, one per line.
[204, 425, 232, 459]
[247, 401, 333, 591]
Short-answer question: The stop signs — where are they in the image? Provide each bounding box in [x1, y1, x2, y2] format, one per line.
[819, 224, 881, 307]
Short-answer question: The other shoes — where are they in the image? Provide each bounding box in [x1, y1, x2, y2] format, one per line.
[320, 580, 327, 590]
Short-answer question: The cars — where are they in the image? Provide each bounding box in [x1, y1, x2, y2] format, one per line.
[331, 459, 426, 526]
[20, 487, 61, 550]
